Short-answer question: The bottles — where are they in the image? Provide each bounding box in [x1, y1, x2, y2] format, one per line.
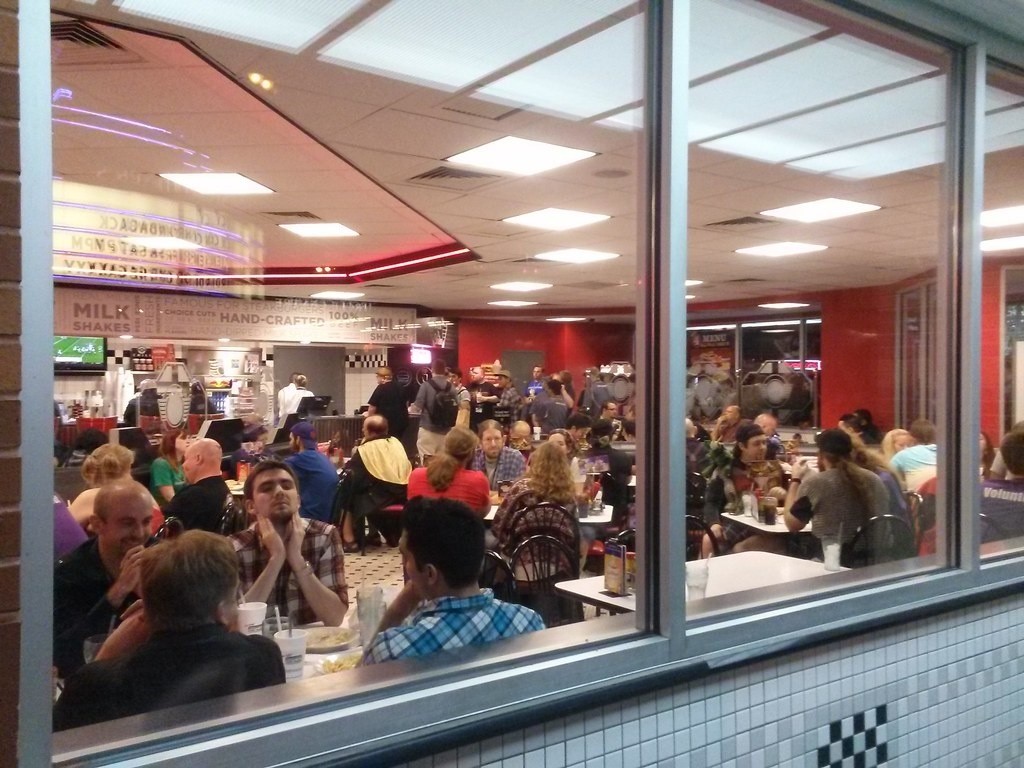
[207, 391, 229, 411]
[624, 551, 636, 586]
[477, 391, 482, 403]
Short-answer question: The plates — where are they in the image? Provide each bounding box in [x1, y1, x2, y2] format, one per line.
[303, 626, 360, 655]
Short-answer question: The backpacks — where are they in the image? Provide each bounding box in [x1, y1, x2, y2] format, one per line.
[427, 378, 459, 429]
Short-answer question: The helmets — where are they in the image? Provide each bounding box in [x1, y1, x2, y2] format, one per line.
[140, 378, 158, 397]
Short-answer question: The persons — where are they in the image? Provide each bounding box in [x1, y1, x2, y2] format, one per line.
[523, 364, 781, 579]
[58, 349, 62, 355]
[54, 370, 314, 429]
[784, 409, 1024, 570]
[35, 429, 542, 730]
[73, 342, 103, 354]
[282, 359, 535, 552]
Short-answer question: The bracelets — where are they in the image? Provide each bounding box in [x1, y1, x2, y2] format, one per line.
[293, 560, 310, 573]
[792, 478, 801, 483]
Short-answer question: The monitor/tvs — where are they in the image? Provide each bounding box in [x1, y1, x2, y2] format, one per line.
[54, 335, 108, 376]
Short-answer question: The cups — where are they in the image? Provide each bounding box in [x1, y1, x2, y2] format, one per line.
[261, 616, 294, 641]
[685, 563, 709, 591]
[274, 629, 308, 683]
[356, 586, 386, 653]
[576, 492, 589, 518]
[741, 488, 778, 526]
[533, 427, 541, 440]
[821, 535, 842, 571]
[100, 367, 134, 422]
[237, 601, 268, 637]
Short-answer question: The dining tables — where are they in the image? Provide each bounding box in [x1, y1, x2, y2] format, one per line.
[483, 506, 614, 525]
[270, 617, 367, 683]
[722, 506, 816, 538]
[554, 550, 850, 617]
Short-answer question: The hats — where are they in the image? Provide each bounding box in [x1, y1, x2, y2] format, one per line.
[735, 423, 765, 442]
[815, 428, 852, 455]
[378, 368, 393, 377]
[586, 420, 619, 447]
[289, 422, 319, 451]
[494, 370, 512, 383]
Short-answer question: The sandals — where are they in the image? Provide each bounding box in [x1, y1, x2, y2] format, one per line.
[365, 533, 383, 547]
[341, 539, 359, 553]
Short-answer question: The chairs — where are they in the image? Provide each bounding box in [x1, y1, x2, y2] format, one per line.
[145, 517, 183, 547]
[218, 500, 247, 537]
[685, 473, 719, 565]
[325, 470, 356, 534]
[482, 500, 583, 627]
